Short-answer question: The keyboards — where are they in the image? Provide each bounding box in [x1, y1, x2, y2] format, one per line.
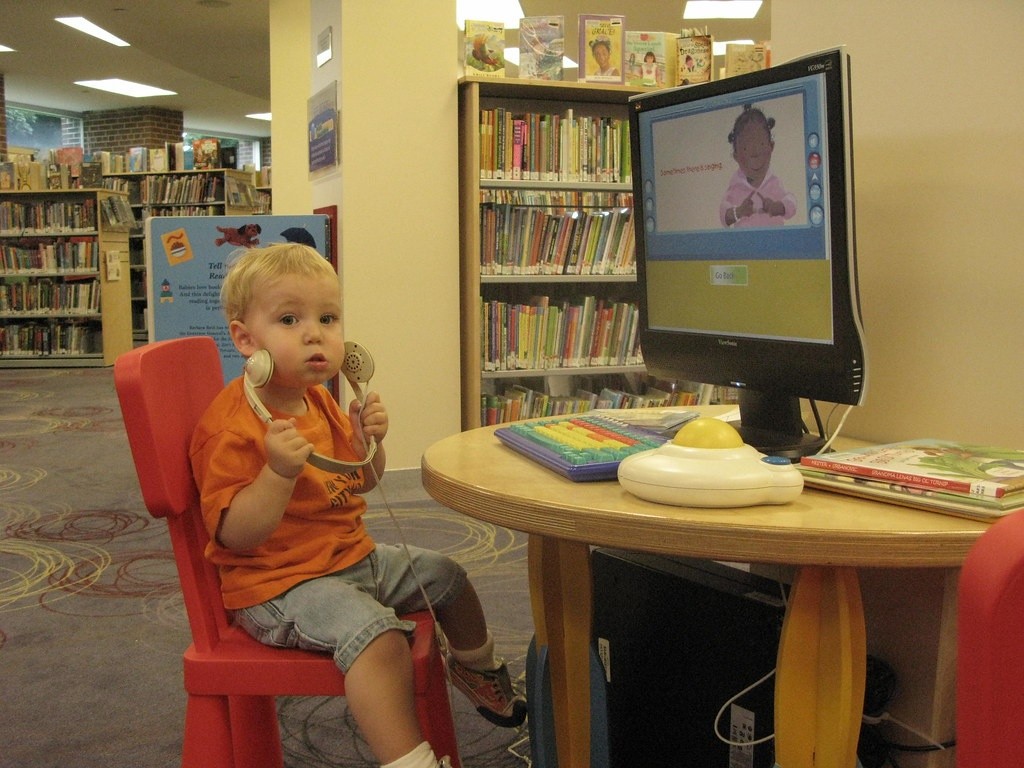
[493, 413, 674, 483]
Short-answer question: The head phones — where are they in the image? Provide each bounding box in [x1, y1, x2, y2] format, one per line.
[243, 340, 376, 474]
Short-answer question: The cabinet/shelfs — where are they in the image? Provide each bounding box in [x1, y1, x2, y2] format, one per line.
[0, 168, 272, 368]
[459, 77, 731, 431]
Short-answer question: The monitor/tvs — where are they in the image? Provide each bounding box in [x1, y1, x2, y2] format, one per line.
[627, 44, 871, 464]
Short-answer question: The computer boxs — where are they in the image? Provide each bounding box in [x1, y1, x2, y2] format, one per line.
[589, 548, 793, 768]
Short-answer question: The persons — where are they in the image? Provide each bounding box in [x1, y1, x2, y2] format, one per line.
[189, 242, 528, 768]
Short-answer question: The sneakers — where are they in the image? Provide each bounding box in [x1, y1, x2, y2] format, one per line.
[445, 653, 527, 727]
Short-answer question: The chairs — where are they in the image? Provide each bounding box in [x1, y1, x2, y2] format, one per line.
[422, 404, 995, 768]
[115, 333, 462, 768]
[958, 509, 1024, 768]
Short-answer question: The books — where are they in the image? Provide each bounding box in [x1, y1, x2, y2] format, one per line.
[480, 107, 743, 425]
[798, 438, 1009, 525]
[0, 139, 273, 356]
